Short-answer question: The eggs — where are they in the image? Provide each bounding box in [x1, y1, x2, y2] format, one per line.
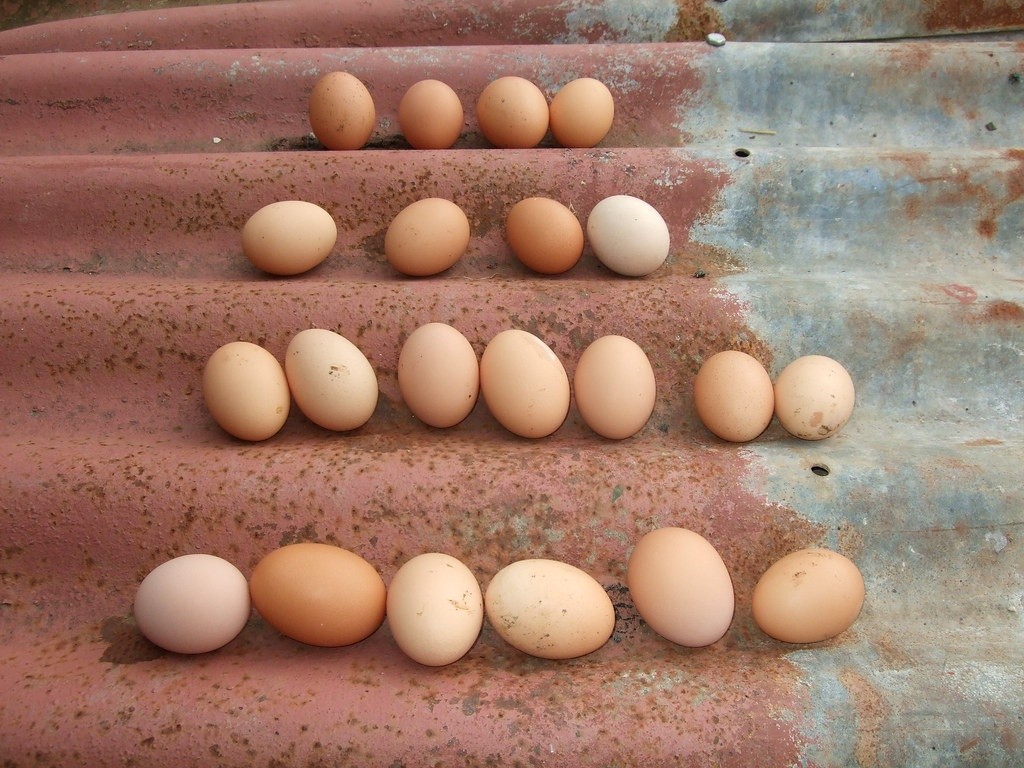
[134, 73, 866, 669]
